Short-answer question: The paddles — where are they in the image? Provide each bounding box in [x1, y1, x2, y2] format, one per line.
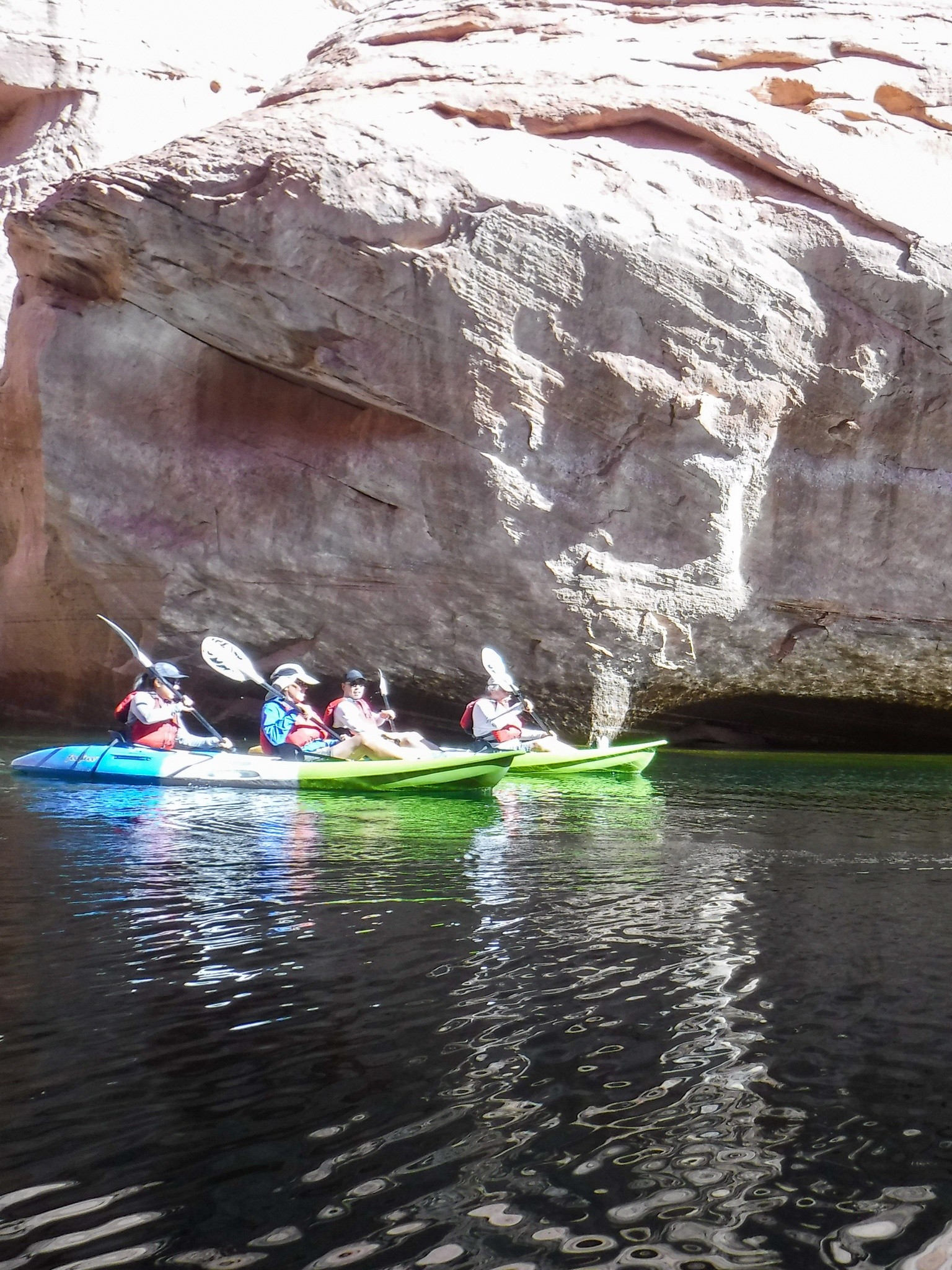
[94, 612, 236, 755]
[376, 667, 400, 745]
[482, 647, 551, 736]
[199, 635, 347, 743]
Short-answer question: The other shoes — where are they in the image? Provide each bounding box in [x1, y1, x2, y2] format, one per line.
[600, 736, 609, 748]
[588, 736, 601, 749]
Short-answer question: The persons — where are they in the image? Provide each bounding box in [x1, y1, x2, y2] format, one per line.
[324, 671, 501, 758]
[116, 662, 236, 754]
[459, 675, 609, 755]
[260, 663, 481, 763]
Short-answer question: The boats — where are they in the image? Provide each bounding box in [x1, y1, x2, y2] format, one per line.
[247, 732, 670, 776]
[5, 742, 527, 798]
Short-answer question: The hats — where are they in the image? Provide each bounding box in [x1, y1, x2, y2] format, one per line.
[487, 673, 520, 692]
[344, 670, 366, 684]
[148, 662, 189, 678]
[270, 664, 320, 685]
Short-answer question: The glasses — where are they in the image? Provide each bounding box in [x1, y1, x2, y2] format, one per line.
[344, 680, 366, 687]
[167, 678, 181, 685]
